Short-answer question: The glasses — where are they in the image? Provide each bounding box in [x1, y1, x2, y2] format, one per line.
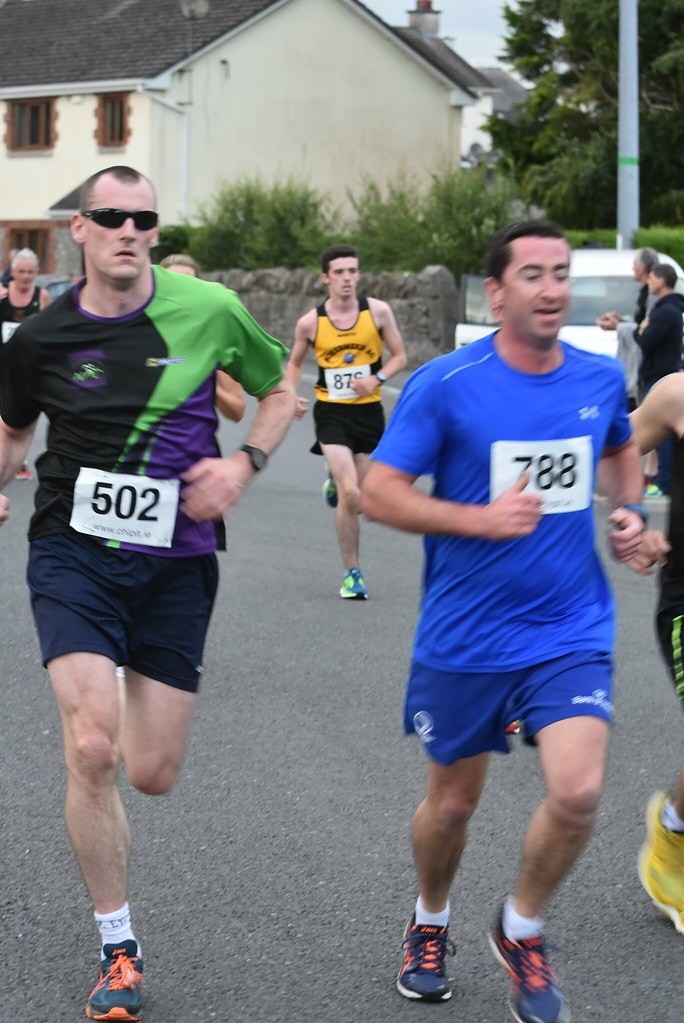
[80, 208, 160, 232]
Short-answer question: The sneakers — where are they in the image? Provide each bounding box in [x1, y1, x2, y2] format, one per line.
[339, 567, 370, 599]
[320, 460, 337, 509]
[396, 911, 454, 1000]
[635, 787, 684, 936]
[485, 901, 569, 1023]
[83, 939, 145, 1022]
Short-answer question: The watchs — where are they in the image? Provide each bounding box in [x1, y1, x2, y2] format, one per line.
[237, 443, 270, 473]
[375, 371, 388, 385]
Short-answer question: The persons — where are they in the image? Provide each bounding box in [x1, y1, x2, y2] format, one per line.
[597, 363, 682, 933]
[597, 264, 683, 497]
[286, 246, 409, 599]
[157, 253, 248, 552]
[628, 246, 660, 325]
[360, 222, 650, 1022]
[1, 167, 298, 1021]
[0, 249, 18, 281]
[0, 247, 53, 481]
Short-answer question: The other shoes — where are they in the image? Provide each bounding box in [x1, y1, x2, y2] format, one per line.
[641, 484, 670, 500]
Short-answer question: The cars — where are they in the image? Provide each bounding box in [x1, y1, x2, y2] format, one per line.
[456, 248, 684, 402]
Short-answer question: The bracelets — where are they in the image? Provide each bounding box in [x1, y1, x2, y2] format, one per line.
[622, 503, 649, 522]
